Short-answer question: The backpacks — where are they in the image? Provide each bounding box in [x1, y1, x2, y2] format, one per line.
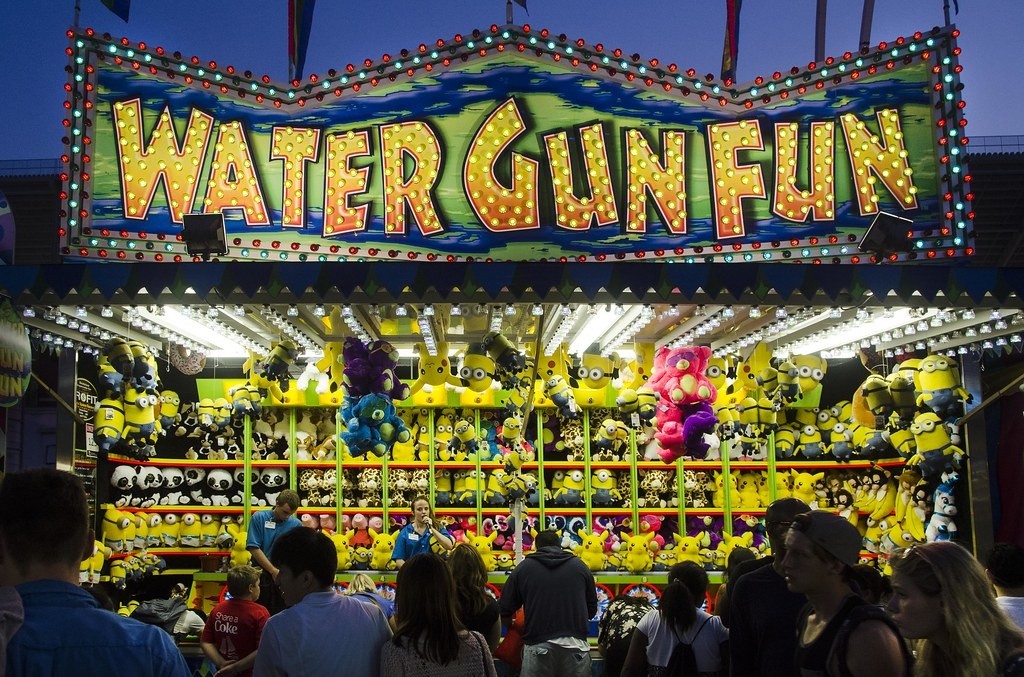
[130, 596, 188, 635]
[666, 615, 713, 676]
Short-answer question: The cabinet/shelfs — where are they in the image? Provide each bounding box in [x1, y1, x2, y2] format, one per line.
[100, 378, 907, 616]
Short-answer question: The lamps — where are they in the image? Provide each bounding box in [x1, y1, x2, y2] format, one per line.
[180, 213, 228, 261]
[858, 211, 915, 258]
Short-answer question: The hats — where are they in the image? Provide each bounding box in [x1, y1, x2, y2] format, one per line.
[789, 509, 864, 569]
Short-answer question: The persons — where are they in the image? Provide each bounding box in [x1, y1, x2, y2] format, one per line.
[391, 496, 455, 571]
[246, 490, 302, 611]
[598, 498, 1024, 677]
[0, 469, 501, 677]
[497, 531, 597, 677]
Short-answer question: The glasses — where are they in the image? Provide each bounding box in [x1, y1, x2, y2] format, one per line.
[901, 543, 933, 567]
[765, 520, 791, 538]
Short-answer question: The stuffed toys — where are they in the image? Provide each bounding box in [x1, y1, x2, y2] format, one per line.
[79, 332, 973, 618]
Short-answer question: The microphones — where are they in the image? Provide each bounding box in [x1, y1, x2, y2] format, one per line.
[422, 515, 433, 534]
